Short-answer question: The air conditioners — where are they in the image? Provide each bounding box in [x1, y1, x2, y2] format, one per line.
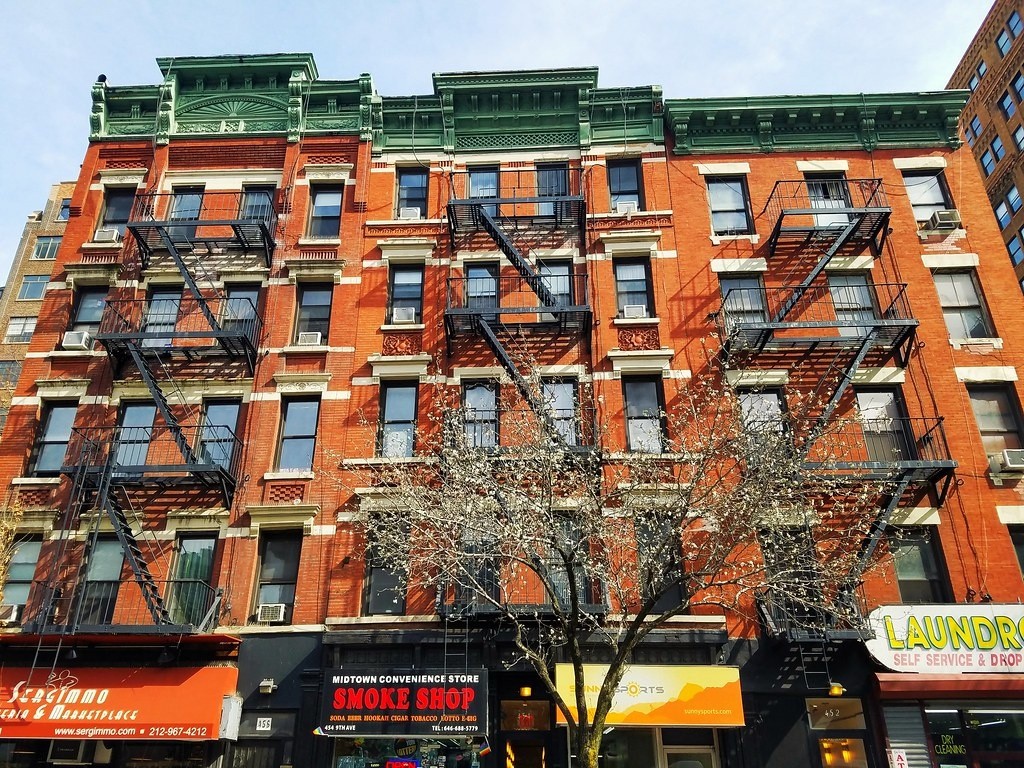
[616, 201, 637, 213]
[399, 207, 421, 219]
[0, 604, 22, 626]
[62, 331, 93, 350]
[928, 209, 962, 229]
[624, 305, 647, 318]
[94, 229, 120, 243]
[998, 449, 1024, 472]
[257, 603, 287, 622]
[393, 307, 416, 325]
[299, 331, 322, 346]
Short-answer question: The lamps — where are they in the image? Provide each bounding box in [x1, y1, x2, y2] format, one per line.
[827, 682, 843, 696]
[258, 678, 277, 695]
